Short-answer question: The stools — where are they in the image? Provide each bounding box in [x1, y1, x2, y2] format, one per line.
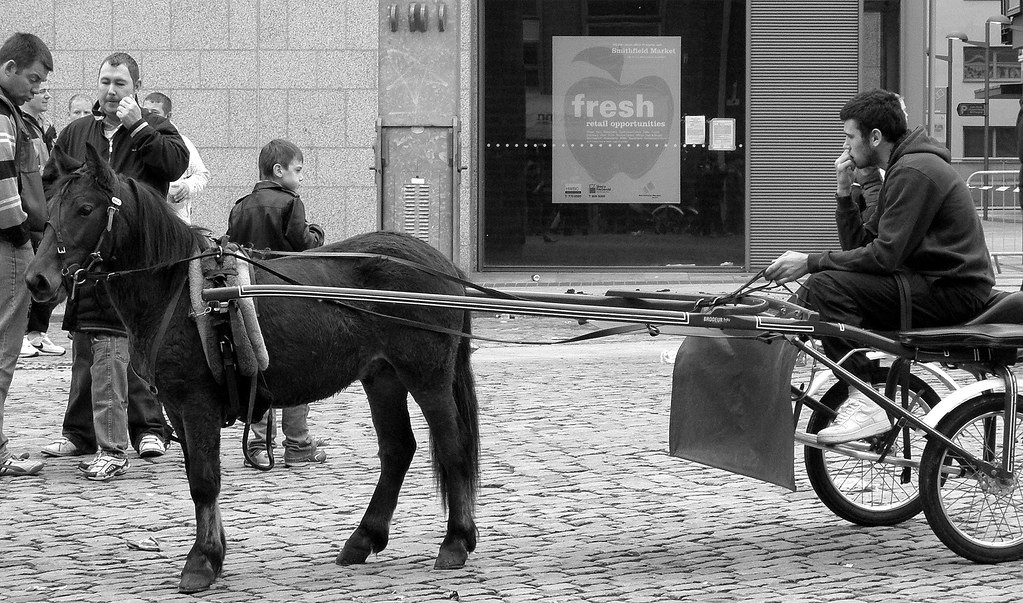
[961, 291, 1023, 364]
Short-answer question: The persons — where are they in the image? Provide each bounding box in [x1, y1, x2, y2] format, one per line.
[0, 34, 53, 478]
[68, 94, 92, 120]
[764, 91, 995, 446]
[850, 90, 907, 226]
[225, 140, 325, 466]
[143, 93, 208, 223]
[42, 53, 211, 456]
[63, 279, 129, 481]
[19, 81, 66, 358]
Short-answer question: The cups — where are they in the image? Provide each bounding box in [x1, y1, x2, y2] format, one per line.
[169, 182, 180, 203]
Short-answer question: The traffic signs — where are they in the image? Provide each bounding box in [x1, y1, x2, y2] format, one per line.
[956, 102, 987, 117]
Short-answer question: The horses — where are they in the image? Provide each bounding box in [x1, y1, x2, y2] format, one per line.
[23, 143, 480, 595]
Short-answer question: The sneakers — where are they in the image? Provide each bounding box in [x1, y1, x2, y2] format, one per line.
[78, 453, 127, 475]
[244, 450, 270, 467]
[85, 454, 130, 480]
[27, 335, 66, 357]
[18, 339, 39, 358]
[140, 435, 165, 456]
[42, 437, 78, 456]
[285, 451, 326, 465]
[817, 394, 892, 443]
[1, 454, 44, 475]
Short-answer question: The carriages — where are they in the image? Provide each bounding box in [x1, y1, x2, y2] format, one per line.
[23, 141, 1023, 593]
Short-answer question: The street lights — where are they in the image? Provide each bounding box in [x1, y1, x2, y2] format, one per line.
[982, 14, 1012, 220]
[946, 31, 969, 166]
